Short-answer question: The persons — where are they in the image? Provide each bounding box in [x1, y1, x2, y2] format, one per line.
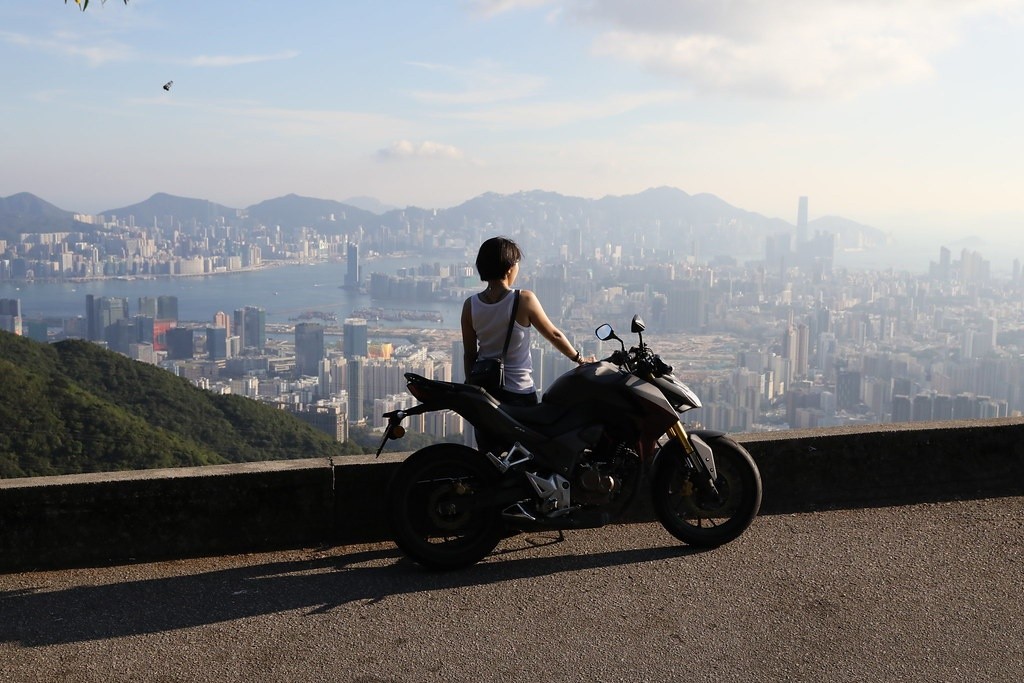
[460, 237, 599, 452]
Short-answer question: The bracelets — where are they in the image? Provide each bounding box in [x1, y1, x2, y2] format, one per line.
[572, 350, 581, 361]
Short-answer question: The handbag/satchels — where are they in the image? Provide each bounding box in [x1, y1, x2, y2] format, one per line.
[464, 351, 506, 394]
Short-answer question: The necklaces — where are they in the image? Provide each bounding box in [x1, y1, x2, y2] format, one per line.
[487, 284, 504, 290]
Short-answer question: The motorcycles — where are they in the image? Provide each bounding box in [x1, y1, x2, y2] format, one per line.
[376, 313, 765, 573]
